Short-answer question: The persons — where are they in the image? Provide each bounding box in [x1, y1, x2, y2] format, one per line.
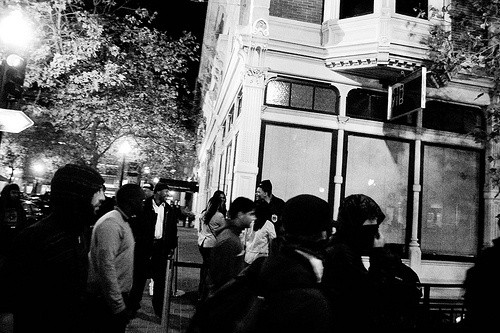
[0, 161, 500, 332]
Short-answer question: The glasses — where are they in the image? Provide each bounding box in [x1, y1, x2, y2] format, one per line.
[219, 197, 225, 199]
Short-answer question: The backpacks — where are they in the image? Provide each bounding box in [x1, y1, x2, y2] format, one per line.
[186, 257, 316, 333]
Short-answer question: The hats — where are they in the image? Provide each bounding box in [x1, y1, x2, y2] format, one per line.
[283, 194, 339, 236]
[257, 180, 272, 197]
[339, 194, 385, 233]
